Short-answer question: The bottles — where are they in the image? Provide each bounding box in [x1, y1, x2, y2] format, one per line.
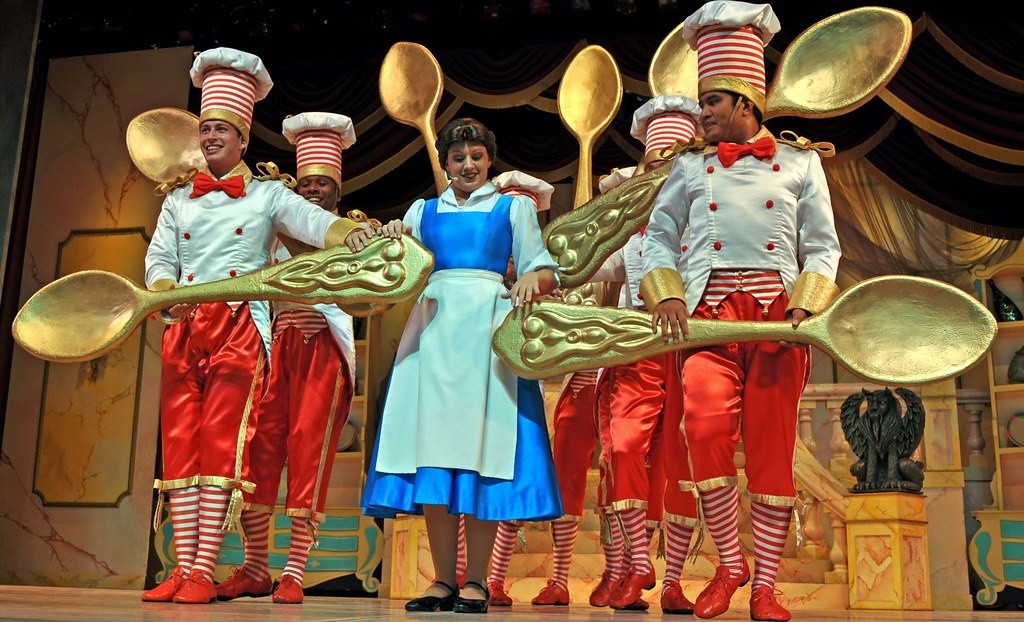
[988, 279, 1024, 323]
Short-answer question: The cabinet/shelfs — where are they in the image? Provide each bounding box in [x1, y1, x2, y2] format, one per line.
[968, 263, 1024, 606]
[153, 316, 384, 592]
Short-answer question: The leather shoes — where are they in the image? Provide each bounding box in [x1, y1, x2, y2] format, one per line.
[404, 578, 459, 613]
[453, 581, 490, 612]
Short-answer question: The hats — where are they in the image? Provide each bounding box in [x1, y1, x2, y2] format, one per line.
[631, 95, 703, 167]
[599, 166, 636, 195]
[282, 111, 356, 195]
[189, 47, 274, 156]
[493, 172, 555, 215]
[682, 1, 782, 122]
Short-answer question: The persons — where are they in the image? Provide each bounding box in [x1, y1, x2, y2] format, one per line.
[535, 96, 708, 617]
[215, 112, 356, 603]
[359, 118, 559, 614]
[456, 170, 553, 607]
[142, 47, 375, 606]
[642, 0, 841, 621]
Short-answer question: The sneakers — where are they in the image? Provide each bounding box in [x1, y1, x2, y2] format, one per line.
[272, 572, 303, 604]
[624, 597, 652, 611]
[173, 568, 218, 604]
[610, 565, 657, 609]
[590, 572, 623, 608]
[694, 552, 750, 618]
[751, 585, 790, 622]
[217, 564, 275, 602]
[659, 579, 696, 614]
[488, 580, 515, 607]
[531, 580, 569, 606]
[141, 566, 189, 603]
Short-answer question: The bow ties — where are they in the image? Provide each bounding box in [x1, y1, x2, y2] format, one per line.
[189, 172, 244, 199]
[718, 137, 777, 167]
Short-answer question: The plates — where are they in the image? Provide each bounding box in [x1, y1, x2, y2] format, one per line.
[1006, 411, 1024, 447]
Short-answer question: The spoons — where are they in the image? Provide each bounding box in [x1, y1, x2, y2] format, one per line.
[537, 6, 912, 291]
[126, 108, 395, 316]
[632, 20, 700, 182]
[557, 44, 624, 310]
[491, 275, 998, 388]
[12, 229, 436, 363]
[379, 41, 449, 198]
